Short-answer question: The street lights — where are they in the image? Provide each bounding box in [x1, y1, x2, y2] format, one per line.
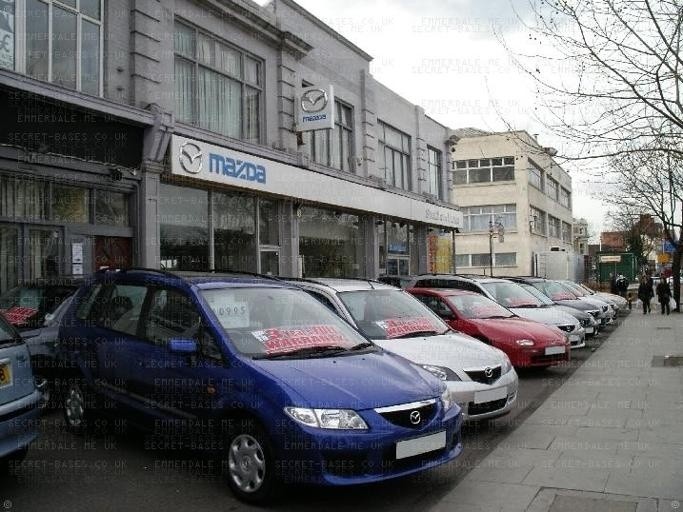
[487, 218, 505, 275]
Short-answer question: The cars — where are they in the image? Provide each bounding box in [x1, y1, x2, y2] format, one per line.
[634, 274, 683, 288]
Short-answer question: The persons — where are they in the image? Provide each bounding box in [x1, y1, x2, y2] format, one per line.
[646, 272, 653, 287]
[656, 276, 673, 315]
[637, 277, 654, 316]
[607, 272, 619, 295]
[615, 272, 629, 297]
